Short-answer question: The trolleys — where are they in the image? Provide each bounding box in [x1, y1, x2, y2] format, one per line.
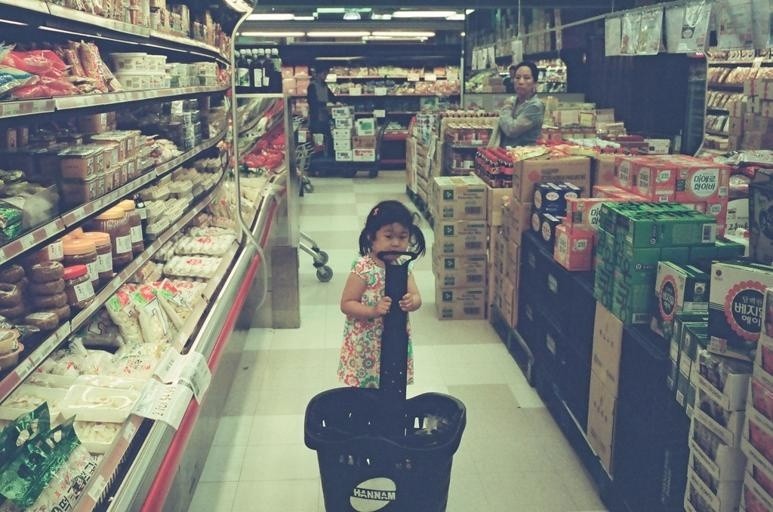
[309, 114, 391, 178]
[295, 140, 317, 194]
[295, 230, 334, 284]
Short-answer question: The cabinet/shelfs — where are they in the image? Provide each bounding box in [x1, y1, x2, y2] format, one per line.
[326, 74, 461, 167]
[0, 0, 277, 512]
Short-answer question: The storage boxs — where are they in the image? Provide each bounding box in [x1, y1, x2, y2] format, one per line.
[328, 101, 383, 165]
[279, 63, 312, 150]
[493, 155, 773, 511]
[430, 173, 515, 322]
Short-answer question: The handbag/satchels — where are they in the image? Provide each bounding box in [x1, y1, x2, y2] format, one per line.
[486, 122, 501, 146]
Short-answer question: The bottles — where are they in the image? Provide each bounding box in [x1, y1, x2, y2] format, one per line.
[232, 48, 282, 93]
[474, 146, 515, 189]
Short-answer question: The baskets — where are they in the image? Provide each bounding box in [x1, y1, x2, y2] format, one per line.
[304, 387, 466, 512]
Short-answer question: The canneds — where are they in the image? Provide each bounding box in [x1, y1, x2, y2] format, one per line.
[54, 227, 113, 310]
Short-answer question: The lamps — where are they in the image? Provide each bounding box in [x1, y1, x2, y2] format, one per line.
[342, 6, 361, 22]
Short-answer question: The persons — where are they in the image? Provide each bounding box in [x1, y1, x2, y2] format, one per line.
[501, 65, 515, 92]
[484, 61, 545, 151]
[336, 200, 426, 389]
[305, 62, 342, 164]
